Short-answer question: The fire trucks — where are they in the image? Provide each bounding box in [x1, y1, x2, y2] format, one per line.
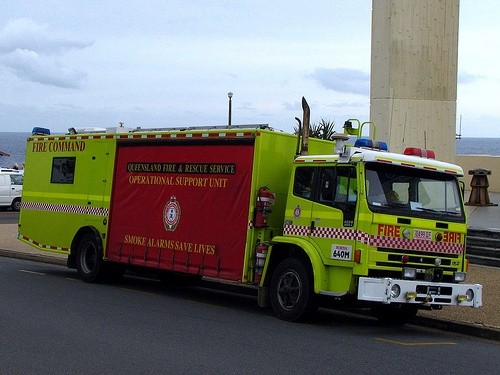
[15, 96, 484, 339]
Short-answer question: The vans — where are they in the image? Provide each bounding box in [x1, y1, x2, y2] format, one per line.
[1, 168, 25, 212]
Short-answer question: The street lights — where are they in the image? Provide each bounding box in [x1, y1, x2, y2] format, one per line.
[227, 91, 233, 125]
